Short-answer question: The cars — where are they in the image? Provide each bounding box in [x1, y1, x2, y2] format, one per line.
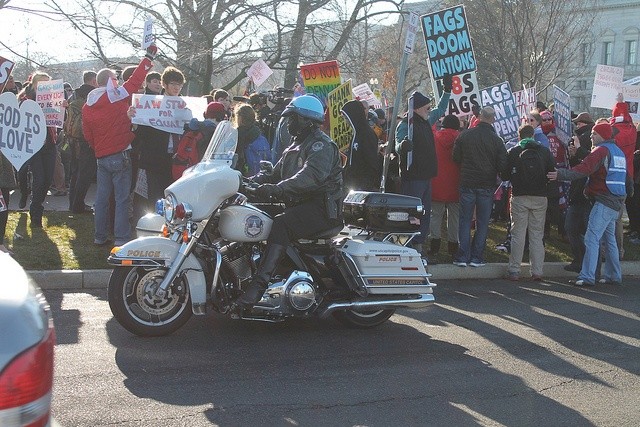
[1, 248, 65, 425]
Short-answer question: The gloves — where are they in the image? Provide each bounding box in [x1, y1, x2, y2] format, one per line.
[145, 41, 157, 62]
[256, 183, 283, 203]
[440, 73, 453, 93]
[399, 136, 414, 153]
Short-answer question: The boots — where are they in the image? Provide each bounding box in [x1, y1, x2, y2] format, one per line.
[448, 242, 458, 257]
[236, 242, 288, 305]
[431, 238, 440, 256]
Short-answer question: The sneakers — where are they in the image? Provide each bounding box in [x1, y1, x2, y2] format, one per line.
[453, 260, 466, 266]
[530, 275, 541, 281]
[469, 257, 488, 266]
[70, 203, 91, 209]
[19, 194, 27, 208]
[598, 276, 622, 285]
[506, 272, 519, 280]
[565, 262, 581, 270]
[419, 251, 432, 264]
[568, 278, 595, 286]
[74, 207, 93, 213]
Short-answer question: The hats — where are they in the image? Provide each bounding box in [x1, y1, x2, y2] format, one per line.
[368, 108, 378, 119]
[571, 112, 594, 123]
[412, 91, 430, 110]
[204, 102, 231, 123]
[441, 115, 460, 130]
[592, 123, 612, 140]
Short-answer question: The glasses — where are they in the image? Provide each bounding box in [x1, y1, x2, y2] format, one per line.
[170, 82, 182, 88]
[541, 118, 552, 121]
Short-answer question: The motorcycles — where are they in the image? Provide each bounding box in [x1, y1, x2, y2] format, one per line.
[106, 119, 439, 338]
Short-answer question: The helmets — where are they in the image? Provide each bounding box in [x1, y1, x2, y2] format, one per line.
[280, 95, 325, 123]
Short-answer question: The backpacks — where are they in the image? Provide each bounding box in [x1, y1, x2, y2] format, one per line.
[510, 141, 546, 194]
[63, 90, 87, 142]
[169, 129, 205, 181]
[242, 136, 270, 177]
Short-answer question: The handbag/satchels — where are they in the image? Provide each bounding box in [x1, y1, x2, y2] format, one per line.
[54, 158, 65, 186]
[131, 129, 147, 155]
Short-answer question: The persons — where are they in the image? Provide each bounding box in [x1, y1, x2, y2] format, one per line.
[452, 106, 508, 267]
[234, 51, 302, 176]
[234, 95, 344, 309]
[0, 46, 233, 246]
[430, 115, 463, 257]
[496, 101, 640, 286]
[394, 91, 438, 263]
[340, 98, 394, 192]
